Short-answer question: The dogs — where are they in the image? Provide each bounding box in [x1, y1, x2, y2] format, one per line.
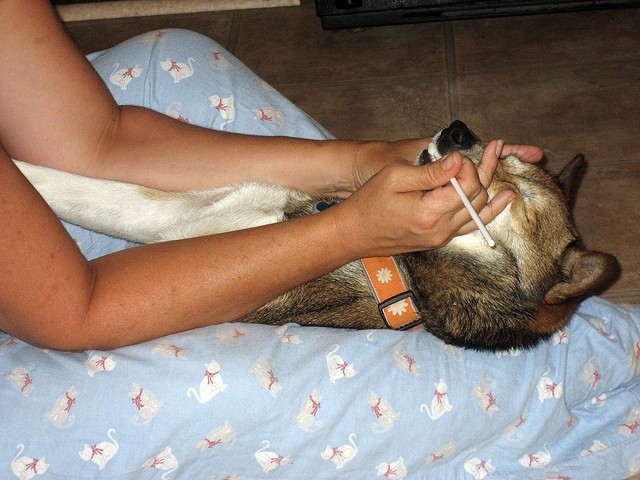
[11, 120, 622, 355]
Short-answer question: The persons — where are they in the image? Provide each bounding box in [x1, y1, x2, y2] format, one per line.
[0, 2, 543, 352]
